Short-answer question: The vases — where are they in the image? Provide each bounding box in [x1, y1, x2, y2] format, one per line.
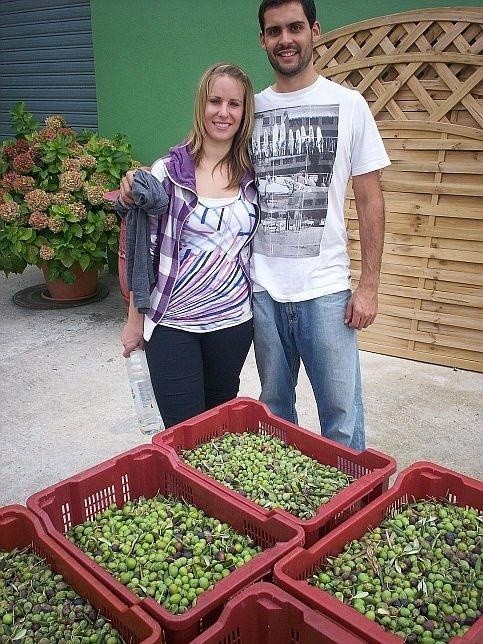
[37, 255, 99, 301]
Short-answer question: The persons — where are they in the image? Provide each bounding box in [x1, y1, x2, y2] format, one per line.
[119, 62, 262, 428]
[117, 0, 391, 455]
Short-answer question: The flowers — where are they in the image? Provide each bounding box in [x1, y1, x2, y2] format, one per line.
[0, 99, 144, 287]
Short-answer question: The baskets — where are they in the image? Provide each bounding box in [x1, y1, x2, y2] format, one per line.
[270, 460, 482, 643]
[187, 580, 368, 643]
[25, 443, 307, 644]
[150, 396, 396, 548]
[0, 503, 165, 643]
